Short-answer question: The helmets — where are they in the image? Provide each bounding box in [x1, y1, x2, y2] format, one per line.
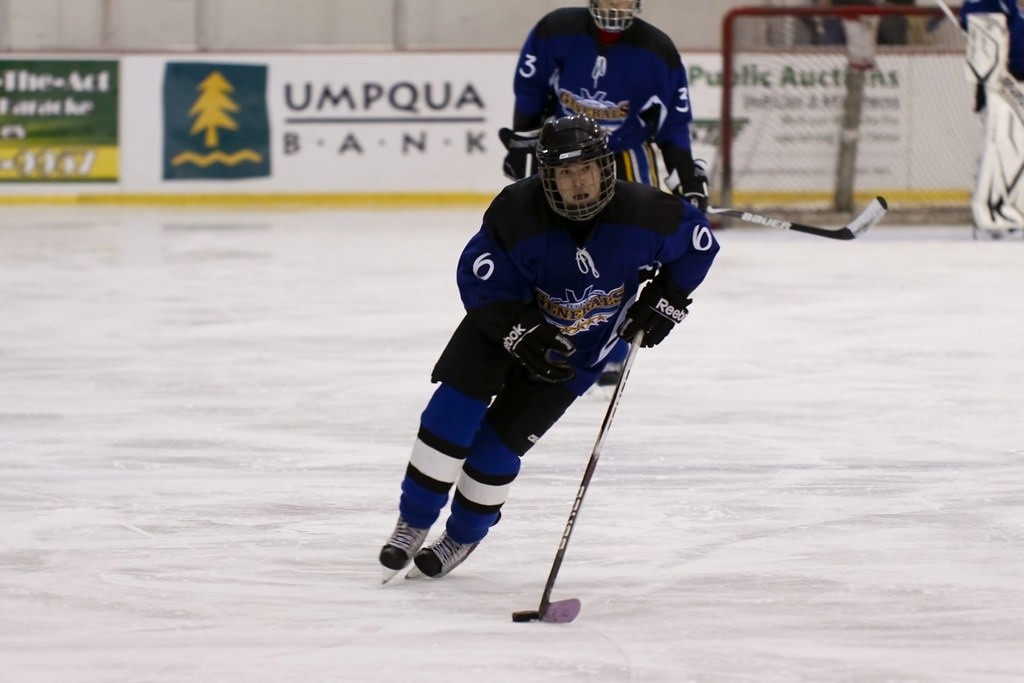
[536, 113, 612, 209]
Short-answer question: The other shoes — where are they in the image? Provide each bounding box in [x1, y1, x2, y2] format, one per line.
[597, 360, 623, 385]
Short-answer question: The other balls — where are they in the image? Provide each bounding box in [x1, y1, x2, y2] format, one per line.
[512, 610, 539, 622]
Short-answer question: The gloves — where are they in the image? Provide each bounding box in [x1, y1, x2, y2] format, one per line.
[500, 312, 577, 384]
[618, 268, 692, 348]
[664, 159, 709, 214]
[498, 128, 541, 181]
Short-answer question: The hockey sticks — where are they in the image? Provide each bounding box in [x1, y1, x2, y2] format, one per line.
[707, 195, 889, 241]
[937, 0, 1024, 110]
[539, 328, 647, 624]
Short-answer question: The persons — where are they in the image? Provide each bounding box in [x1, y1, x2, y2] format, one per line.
[499, 0, 709, 386]
[958, 0, 1024, 242]
[378, 112, 721, 587]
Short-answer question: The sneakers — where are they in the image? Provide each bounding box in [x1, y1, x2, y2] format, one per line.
[379, 515, 430, 584]
[405, 530, 480, 581]
